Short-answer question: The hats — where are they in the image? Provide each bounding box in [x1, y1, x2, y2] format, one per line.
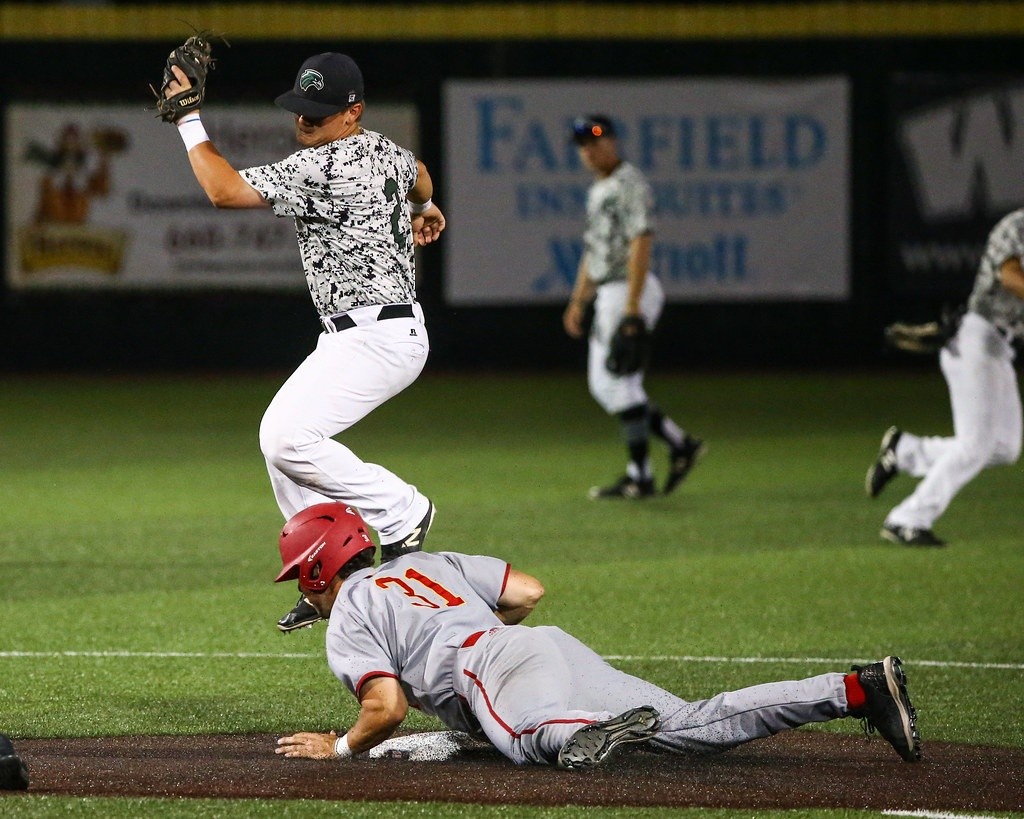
[570, 114, 614, 146]
[274, 53, 364, 118]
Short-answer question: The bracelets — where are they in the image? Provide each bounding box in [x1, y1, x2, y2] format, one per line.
[177, 112, 210, 152]
[407, 198, 432, 214]
[334, 733, 353, 758]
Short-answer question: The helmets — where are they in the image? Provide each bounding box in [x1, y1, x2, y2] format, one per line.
[274, 501, 376, 591]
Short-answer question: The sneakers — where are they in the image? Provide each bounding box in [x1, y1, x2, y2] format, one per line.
[880, 524, 943, 548]
[557, 705, 663, 770]
[663, 432, 703, 495]
[850, 656, 921, 764]
[865, 425, 902, 498]
[589, 474, 655, 501]
[277, 594, 324, 635]
[380, 499, 436, 565]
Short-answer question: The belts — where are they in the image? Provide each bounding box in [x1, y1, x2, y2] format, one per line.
[456, 630, 493, 745]
[997, 324, 1016, 348]
[321, 304, 415, 334]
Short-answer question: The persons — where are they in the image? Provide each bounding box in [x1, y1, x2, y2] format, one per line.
[865, 208, 1024, 548]
[275, 503, 923, 770]
[0, 731, 30, 792]
[562, 113, 706, 500]
[156, 38, 446, 635]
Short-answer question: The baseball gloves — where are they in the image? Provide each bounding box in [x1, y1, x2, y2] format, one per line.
[606, 312, 649, 377]
[884, 312, 957, 353]
[142, 15, 232, 124]
